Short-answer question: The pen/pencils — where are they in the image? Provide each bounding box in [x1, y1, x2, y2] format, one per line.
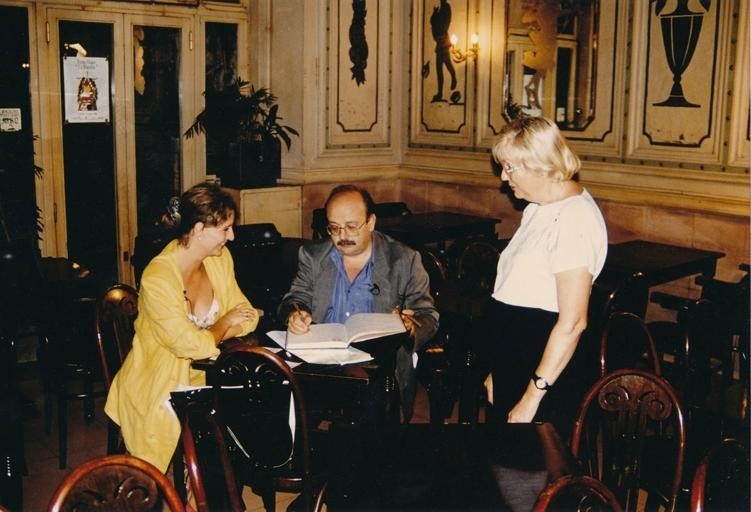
[293, 301, 312, 333]
[395, 305, 422, 328]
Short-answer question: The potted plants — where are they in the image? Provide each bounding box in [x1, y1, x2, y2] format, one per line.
[182, 78, 300, 187]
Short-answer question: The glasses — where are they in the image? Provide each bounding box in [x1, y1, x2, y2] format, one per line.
[326, 220, 367, 236]
[503, 163, 521, 174]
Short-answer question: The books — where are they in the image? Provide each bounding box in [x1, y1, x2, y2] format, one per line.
[283, 312, 409, 351]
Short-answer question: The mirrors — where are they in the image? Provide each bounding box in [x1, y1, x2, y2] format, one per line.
[501, 0, 600, 132]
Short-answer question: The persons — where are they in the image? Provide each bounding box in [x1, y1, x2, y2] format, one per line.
[277, 186, 440, 428]
[482, 118, 610, 424]
[103, 182, 259, 510]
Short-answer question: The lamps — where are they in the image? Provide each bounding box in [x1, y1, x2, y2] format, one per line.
[450, 33, 479, 63]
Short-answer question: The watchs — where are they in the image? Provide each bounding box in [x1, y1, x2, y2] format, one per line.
[529, 370, 554, 393]
